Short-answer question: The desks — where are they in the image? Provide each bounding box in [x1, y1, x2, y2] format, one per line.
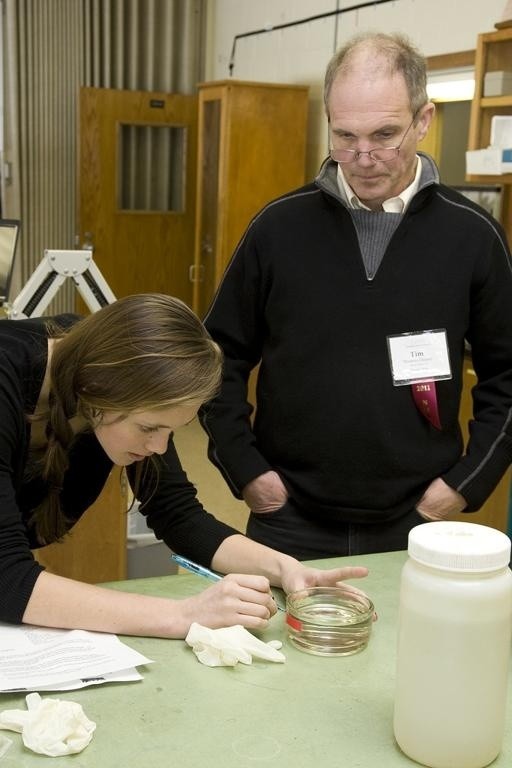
[0, 550, 511, 768]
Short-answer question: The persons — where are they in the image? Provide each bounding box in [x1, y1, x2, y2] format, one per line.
[198, 28, 512, 562]
[0, 292, 380, 642]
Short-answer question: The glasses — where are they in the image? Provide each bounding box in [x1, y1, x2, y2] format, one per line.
[327, 108, 420, 164]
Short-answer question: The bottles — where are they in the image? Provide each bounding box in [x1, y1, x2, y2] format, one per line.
[392, 520, 510, 768]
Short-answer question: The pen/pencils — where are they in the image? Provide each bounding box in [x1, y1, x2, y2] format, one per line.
[168, 551, 287, 612]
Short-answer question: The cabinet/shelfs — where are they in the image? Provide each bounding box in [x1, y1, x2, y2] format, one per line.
[191, 80, 312, 417]
[465, 25, 512, 184]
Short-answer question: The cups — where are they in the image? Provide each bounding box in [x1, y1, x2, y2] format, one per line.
[283, 588, 375, 656]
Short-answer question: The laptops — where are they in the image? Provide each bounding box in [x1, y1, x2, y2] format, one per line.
[0, 217, 21, 304]
